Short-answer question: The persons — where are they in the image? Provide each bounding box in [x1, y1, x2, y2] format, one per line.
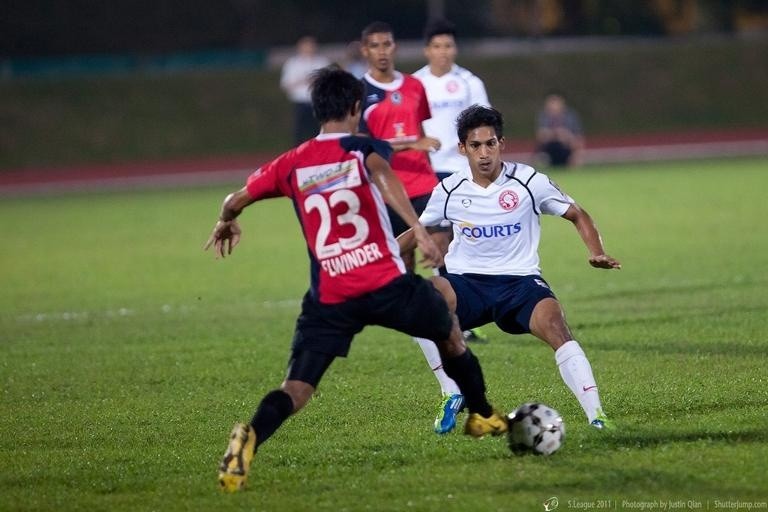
[341, 35, 371, 80]
[202, 64, 509, 494]
[277, 35, 337, 146]
[353, 22, 492, 344]
[387, 103, 622, 433]
[534, 90, 583, 171]
[408, 28, 494, 336]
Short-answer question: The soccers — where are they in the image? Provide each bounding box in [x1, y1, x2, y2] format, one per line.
[507, 403, 564, 457]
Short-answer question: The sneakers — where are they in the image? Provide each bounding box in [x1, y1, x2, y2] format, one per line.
[218, 423, 257, 495]
[462, 330, 489, 344]
[468, 408, 509, 437]
[592, 416, 612, 431]
[433, 393, 466, 435]
